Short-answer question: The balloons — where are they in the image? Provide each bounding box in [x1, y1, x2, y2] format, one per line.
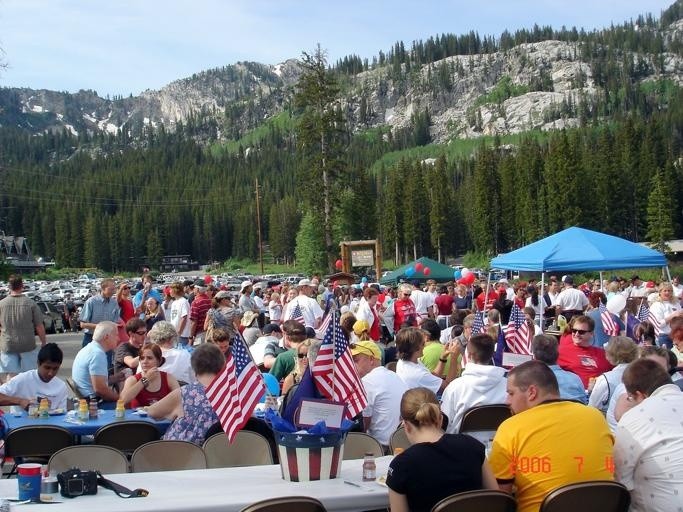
[359, 276, 369, 288]
[404, 263, 429, 277]
[204, 275, 212, 283]
[454, 267, 475, 285]
[336, 260, 342, 269]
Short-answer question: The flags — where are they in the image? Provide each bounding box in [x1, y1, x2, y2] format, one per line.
[598, 299, 619, 336]
[624, 311, 641, 344]
[638, 301, 661, 341]
[505, 302, 535, 357]
[458, 309, 488, 369]
[204, 327, 268, 447]
[289, 303, 306, 326]
[311, 312, 368, 421]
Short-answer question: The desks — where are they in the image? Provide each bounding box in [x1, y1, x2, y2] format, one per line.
[5, 406, 275, 442]
[0, 455, 391, 512]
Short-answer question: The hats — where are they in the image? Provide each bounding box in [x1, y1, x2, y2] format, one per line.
[213, 291, 234, 300]
[189, 279, 207, 288]
[426, 279, 436, 284]
[261, 324, 282, 334]
[629, 275, 639, 282]
[352, 319, 369, 334]
[240, 311, 258, 326]
[499, 288, 506, 294]
[298, 278, 316, 287]
[239, 281, 252, 292]
[645, 281, 655, 288]
[285, 323, 306, 335]
[349, 341, 381, 360]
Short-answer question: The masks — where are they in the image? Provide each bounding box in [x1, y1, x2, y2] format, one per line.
[564, 276, 572, 283]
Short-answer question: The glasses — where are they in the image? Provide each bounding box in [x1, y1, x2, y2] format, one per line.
[133, 329, 146, 335]
[625, 394, 632, 401]
[519, 288, 523, 291]
[123, 286, 130, 289]
[297, 352, 307, 358]
[403, 293, 411, 296]
[349, 343, 374, 355]
[571, 328, 591, 334]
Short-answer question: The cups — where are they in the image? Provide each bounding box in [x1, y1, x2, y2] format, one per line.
[18, 476, 42, 503]
[17, 463, 42, 476]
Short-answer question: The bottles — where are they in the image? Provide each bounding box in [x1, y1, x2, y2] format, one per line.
[363, 452, 376, 482]
[271, 395, 278, 411]
[265, 394, 271, 408]
[79, 399, 88, 422]
[588, 377, 595, 397]
[553, 320, 556, 332]
[115, 400, 125, 418]
[29, 401, 35, 416]
[39, 398, 48, 419]
[89, 399, 98, 421]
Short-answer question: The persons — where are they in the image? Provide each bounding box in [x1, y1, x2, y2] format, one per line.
[312, 273, 363, 312]
[148, 342, 228, 446]
[445, 313, 475, 347]
[118, 343, 180, 410]
[667, 349, 683, 392]
[440, 309, 466, 345]
[0, 342, 74, 416]
[410, 278, 499, 315]
[670, 326, 683, 368]
[384, 386, 499, 512]
[636, 321, 656, 346]
[221, 284, 227, 291]
[161, 286, 176, 321]
[532, 334, 587, 405]
[264, 280, 289, 319]
[238, 281, 260, 316]
[612, 358, 683, 512]
[284, 279, 324, 329]
[116, 283, 136, 324]
[137, 320, 197, 386]
[649, 281, 681, 347]
[487, 358, 616, 512]
[603, 345, 670, 436]
[205, 284, 220, 299]
[264, 319, 299, 370]
[268, 322, 308, 384]
[281, 337, 320, 395]
[369, 284, 394, 340]
[113, 318, 148, 394]
[185, 279, 211, 352]
[168, 282, 191, 345]
[212, 328, 233, 361]
[439, 333, 510, 449]
[489, 278, 524, 325]
[287, 288, 299, 301]
[395, 327, 461, 397]
[543, 278, 559, 329]
[248, 323, 283, 368]
[64, 292, 78, 328]
[241, 311, 263, 347]
[143, 297, 166, 332]
[203, 290, 240, 344]
[601, 275, 659, 299]
[0, 273, 46, 373]
[523, 307, 536, 320]
[71, 321, 127, 410]
[557, 276, 589, 322]
[671, 275, 683, 297]
[580, 278, 601, 294]
[348, 340, 407, 458]
[182, 280, 195, 306]
[132, 273, 162, 320]
[419, 319, 461, 380]
[588, 336, 639, 414]
[394, 284, 421, 333]
[352, 320, 374, 342]
[339, 310, 360, 344]
[585, 291, 625, 347]
[357, 287, 381, 342]
[215, 275, 222, 288]
[254, 287, 269, 311]
[557, 315, 615, 405]
[79, 278, 120, 372]
[524, 285, 548, 333]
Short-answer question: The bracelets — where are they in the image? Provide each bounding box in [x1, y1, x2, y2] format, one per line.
[439, 358, 447, 363]
[141, 377, 149, 387]
[189, 335, 193, 339]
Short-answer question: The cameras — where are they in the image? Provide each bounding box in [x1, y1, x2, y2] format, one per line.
[59, 468, 101, 498]
[448, 335, 470, 354]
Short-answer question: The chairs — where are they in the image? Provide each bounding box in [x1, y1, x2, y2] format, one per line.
[6, 424, 73, 479]
[131, 442, 205, 472]
[205, 429, 276, 465]
[90, 419, 163, 458]
[385, 360, 398, 370]
[46, 446, 129, 474]
[459, 405, 512, 447]
[433, 491, 520, 512]
[240, 497, 329, 512]
[538, 481, 632, 511]
[391, 428, 419, 456]
[342, 432, 386, 460]
